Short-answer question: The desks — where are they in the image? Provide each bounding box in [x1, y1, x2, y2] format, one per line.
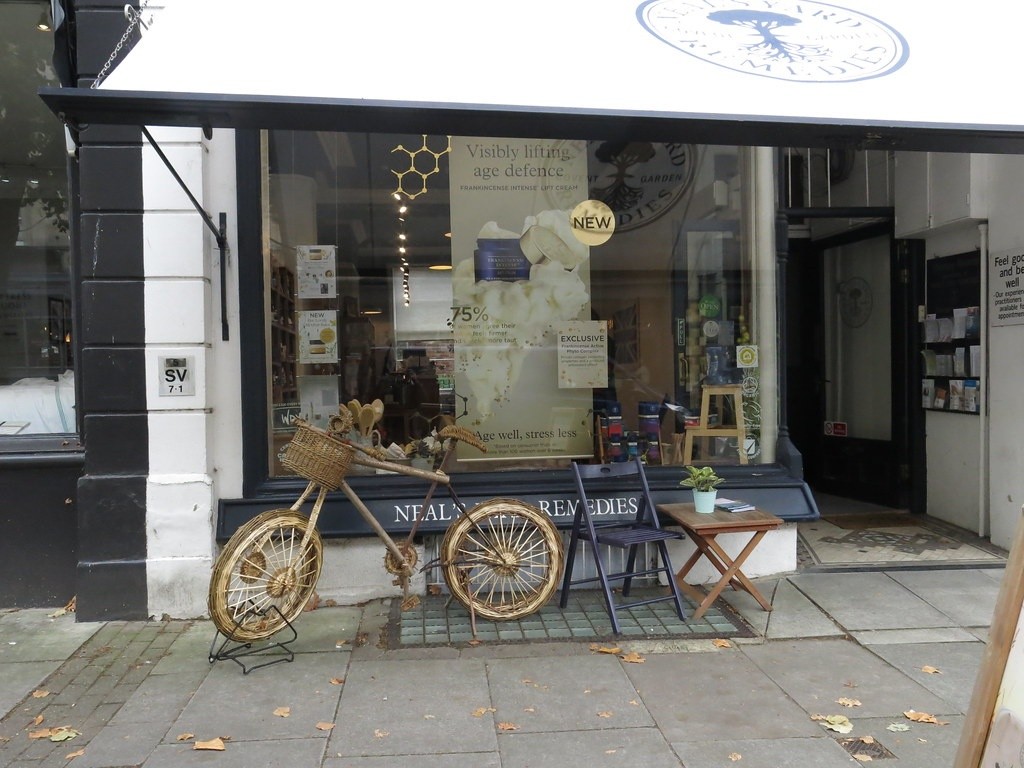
[654, 496, 783, 630]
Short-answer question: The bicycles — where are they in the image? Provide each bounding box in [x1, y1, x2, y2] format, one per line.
[204, 397, 565, 643]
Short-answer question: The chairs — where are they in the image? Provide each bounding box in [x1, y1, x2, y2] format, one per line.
[558, 455, 685, 635]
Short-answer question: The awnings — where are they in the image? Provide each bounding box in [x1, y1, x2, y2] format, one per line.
[41, 0, 1024, 249]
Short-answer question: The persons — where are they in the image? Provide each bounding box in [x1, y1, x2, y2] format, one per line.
[399, 341, 439, 435]
[592, 309, 618, 412]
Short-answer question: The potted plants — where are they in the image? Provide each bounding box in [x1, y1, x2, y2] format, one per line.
[679, 465, 725, 514]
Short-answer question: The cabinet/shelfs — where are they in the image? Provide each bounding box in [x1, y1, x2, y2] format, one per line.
[673, 382, 748, 466]
